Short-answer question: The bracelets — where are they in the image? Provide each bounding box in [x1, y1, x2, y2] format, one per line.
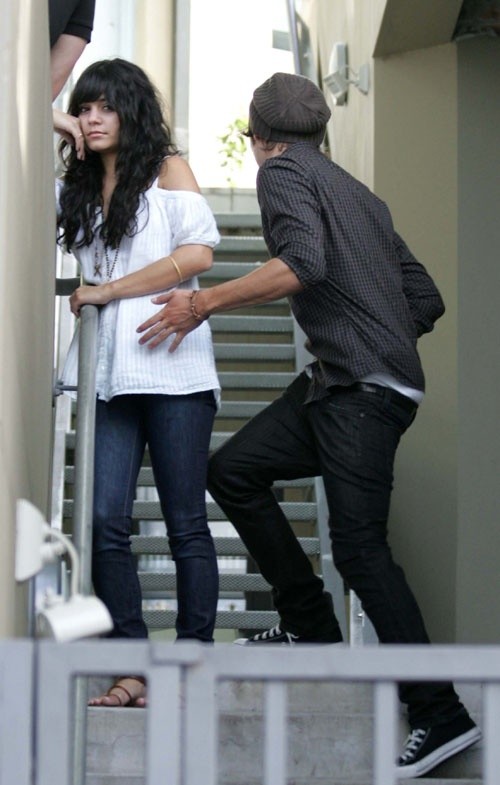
[167, 254, 184, 285]
[190, 289, 210, 320]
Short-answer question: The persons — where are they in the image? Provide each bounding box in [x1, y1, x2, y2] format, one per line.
[207, 72, 481, 780]
[47, 0, 95, 162]
[60, 56, 222, 708]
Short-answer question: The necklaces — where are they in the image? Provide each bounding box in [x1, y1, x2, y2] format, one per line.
[89, 225, 122, 282]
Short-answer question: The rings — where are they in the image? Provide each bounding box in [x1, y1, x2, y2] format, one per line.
[79, 133, 83, 136]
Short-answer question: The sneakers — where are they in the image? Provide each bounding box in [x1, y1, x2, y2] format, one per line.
[232, 626, 346, 649]
[394, 707, 482, 777]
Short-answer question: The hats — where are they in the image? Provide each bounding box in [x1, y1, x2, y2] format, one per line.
[248, 71, 331, 145]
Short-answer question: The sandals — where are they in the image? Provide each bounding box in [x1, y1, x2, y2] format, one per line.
[89, 675, 147, 707]
